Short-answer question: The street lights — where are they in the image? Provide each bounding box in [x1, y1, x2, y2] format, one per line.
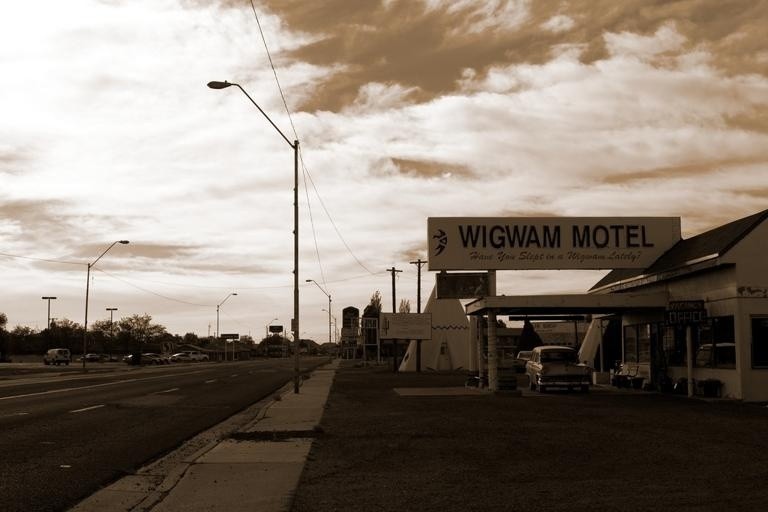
[216, 293, 237, 338]
[306, 279, 332, 344]
[83, 241, 130, 368]
[208, 80, 300, 395]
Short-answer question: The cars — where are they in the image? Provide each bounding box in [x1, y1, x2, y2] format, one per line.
[526, 346, 592, 392]
[81, 351, 209, 366]
[516, 350, 532, 361]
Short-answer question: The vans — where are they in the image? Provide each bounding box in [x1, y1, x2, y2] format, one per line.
[44, 349, 71, 365]
